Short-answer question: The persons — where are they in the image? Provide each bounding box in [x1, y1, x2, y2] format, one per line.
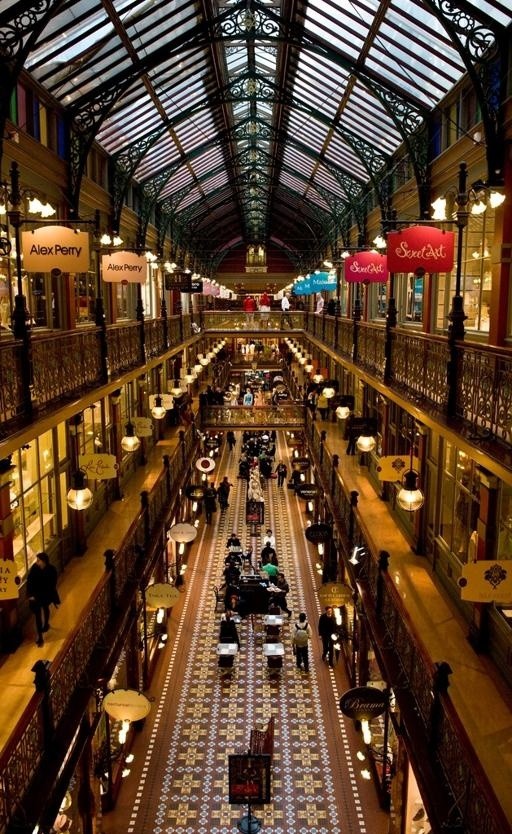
[243, 295, 256, 328]
[259, 291, 270, 327]
[280, 292, 293, 330]
[26, 552, 61, 644]
[204, 385, 338, 673]
[316, 292, 324, 314]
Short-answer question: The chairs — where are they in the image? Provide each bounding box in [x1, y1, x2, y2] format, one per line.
[211, 585, 284, 669]
[227, 549, 277, 574]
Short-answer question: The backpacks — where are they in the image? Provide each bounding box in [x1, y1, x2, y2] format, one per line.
[295, 623, 308, 647]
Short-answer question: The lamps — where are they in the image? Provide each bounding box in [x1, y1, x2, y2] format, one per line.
[283, 335, 425, 516]
[337, 181, 506, 261]
[65, 333, 226, 511]
[12, 189, 160, 271]
[330, 627, 357, 652]
[144, 634, 170, 649]
